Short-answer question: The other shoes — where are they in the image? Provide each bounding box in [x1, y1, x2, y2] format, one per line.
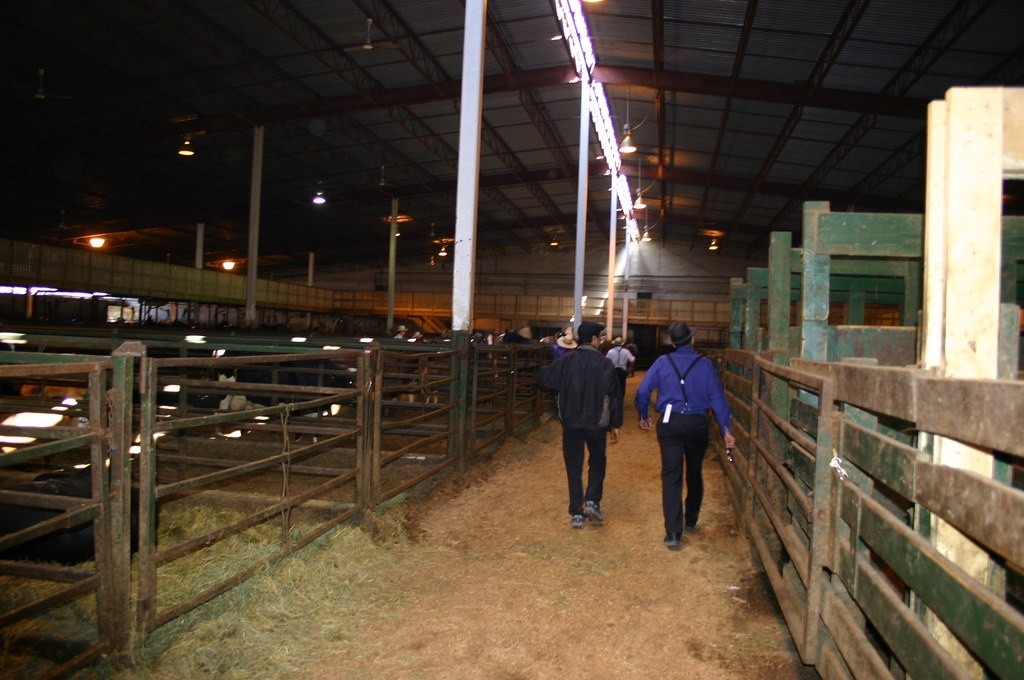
[583, 500, 603, 526]
[572, 514, 586, 529]
[663, 532, 681, 546]
[685, 525, 696, 533]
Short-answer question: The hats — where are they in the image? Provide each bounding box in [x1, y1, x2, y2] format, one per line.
[556, 335, 579, 349]
[578, 321, 606, 337]
[471, 332, 482, 337]
[612, 337, 625, 346]
[413, 332, 424, 337]
[396, 325, 409, 332]
[666, 320, 696, 344]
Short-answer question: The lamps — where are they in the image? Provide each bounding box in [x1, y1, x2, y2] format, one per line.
[633, 155, 648, 210]
[619, 87, 637, 154]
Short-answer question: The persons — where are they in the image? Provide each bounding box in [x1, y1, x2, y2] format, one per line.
[413, 332, 423, 338]
[393, 325, 408, 339]
[551, 332, 568, 360]
[605, 337, 635, 425]
[625, 339, 638, 377]
[539, 321, 623, 527]
[634, 321, 736, 545]
